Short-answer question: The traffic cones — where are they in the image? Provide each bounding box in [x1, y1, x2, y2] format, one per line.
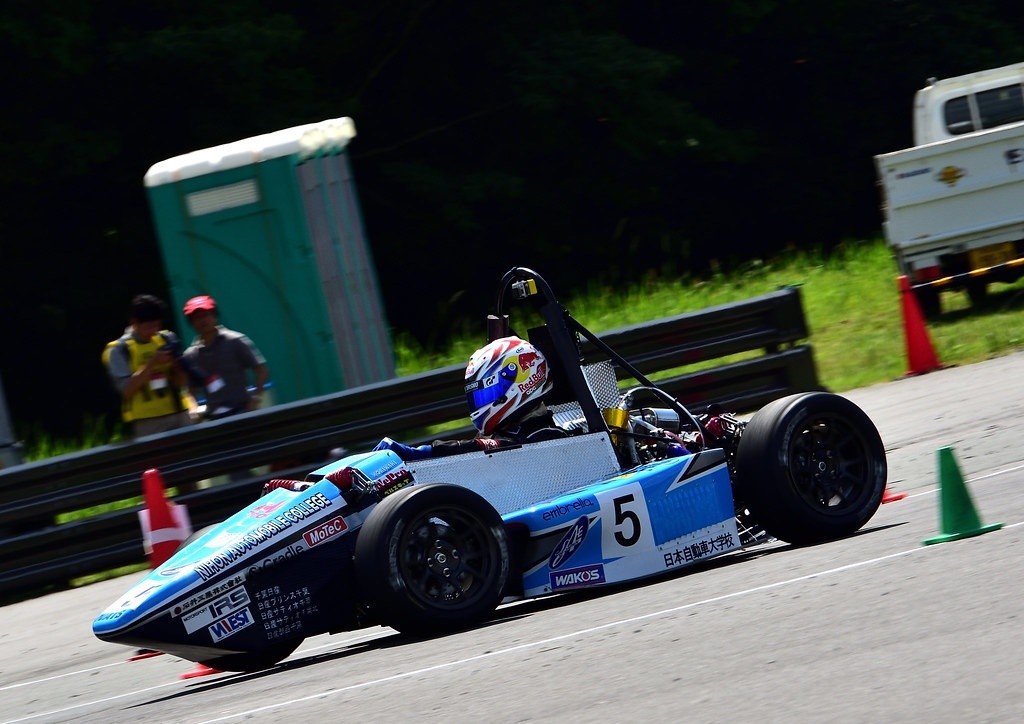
[124, 467, 179, 662]
[923, 444, 1002, 546]
[892, 276, 959, 379]
[183, 658, 225, 680]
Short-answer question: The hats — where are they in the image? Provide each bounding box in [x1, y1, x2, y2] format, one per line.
[183, 296, 216, 316]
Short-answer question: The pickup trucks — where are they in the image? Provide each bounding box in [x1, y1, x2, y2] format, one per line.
[875, 62, 1024, 324]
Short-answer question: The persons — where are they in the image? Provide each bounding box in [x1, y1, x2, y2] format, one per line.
[371, 335, 582, 459]
[99, 294, 268, 500]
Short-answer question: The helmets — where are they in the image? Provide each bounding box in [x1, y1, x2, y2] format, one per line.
[463, 335, 553, 435]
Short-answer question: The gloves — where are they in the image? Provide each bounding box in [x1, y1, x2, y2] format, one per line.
[373, 437, 433, 461]
